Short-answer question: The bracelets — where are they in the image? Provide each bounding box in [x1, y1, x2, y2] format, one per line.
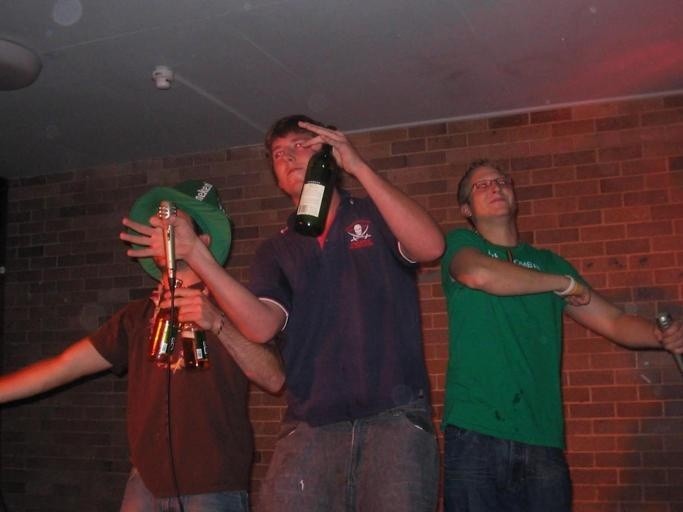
[213, 312, 224, 339]
[570, 281, 584, 297]
[553, 275, 576, 296]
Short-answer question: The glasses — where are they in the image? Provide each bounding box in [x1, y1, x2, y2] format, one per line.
[466, 175, 510, 203]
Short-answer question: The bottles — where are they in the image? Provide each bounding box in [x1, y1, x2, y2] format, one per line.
[145, 278, 184, 366]
[179, 321, 209, 374]
[289, 123, 338, 236]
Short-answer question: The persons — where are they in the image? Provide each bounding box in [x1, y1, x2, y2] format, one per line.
[440, 158, 683, 512]
[120, 113, 445, 511]
[0, 178, 286, 511]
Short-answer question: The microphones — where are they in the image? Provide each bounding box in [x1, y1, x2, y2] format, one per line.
[656, 312, 683, 374]
[158, 200, 175, 289]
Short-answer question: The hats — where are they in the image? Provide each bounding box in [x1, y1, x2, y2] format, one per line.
[126, 178, 232, 284]
